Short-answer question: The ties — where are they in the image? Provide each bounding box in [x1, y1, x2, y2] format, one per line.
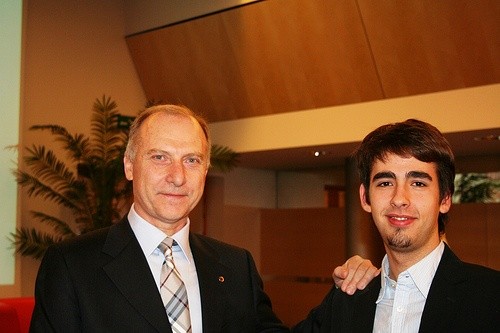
[157, 237, 192, 333]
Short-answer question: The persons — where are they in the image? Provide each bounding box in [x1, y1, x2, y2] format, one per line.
[291, 117, 500, 333]
[29, 104, 384, 333]
